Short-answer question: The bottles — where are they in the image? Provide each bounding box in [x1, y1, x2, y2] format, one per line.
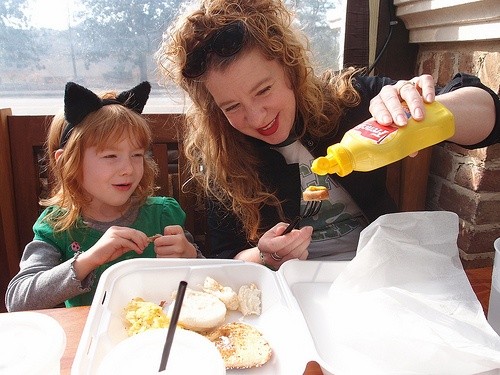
[310, 95, 456, 177]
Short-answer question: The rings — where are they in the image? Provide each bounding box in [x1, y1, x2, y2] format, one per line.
[398, 81, 415, 96]
[270, 252, 282, 261]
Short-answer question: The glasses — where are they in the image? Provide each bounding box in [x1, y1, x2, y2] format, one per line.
[182, 20, 248, 78]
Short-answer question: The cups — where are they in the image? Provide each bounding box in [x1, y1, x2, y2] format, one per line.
[487, 238, 500, 337]
[96, 326, 227, 375]
[0, 311, 68, 375]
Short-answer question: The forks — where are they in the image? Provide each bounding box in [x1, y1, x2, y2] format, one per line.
[279, 201, 323, 236]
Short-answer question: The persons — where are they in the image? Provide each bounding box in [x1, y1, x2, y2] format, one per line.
[5, 81, 207, 313]
[152, 0, 500, 272]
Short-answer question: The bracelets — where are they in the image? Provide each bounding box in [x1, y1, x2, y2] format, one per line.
[260, 251, 278, 271]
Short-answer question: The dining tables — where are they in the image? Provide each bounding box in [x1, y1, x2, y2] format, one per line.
[0, 266, 493, 375]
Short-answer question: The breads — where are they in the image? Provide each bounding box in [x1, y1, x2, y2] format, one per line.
[121, 276, 270, 370]
[303, 186, 329, 201]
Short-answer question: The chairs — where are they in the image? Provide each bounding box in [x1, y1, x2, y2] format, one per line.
[0, 108, 208, 281]
